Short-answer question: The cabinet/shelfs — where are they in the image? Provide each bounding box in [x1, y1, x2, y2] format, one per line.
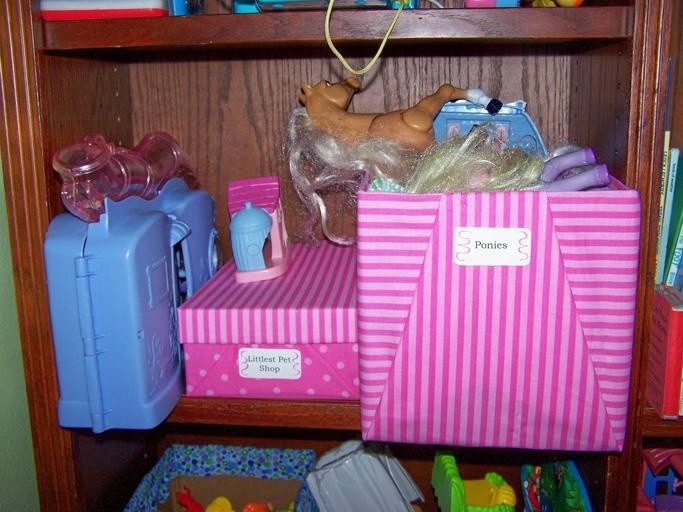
[0, 1, 683, 510]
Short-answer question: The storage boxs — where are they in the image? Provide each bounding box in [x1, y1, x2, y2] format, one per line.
[357, 158, 640, 454]
[177, 238, 361, 400]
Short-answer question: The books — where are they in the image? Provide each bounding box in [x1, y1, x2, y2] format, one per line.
[651, 126, 683, 293]
